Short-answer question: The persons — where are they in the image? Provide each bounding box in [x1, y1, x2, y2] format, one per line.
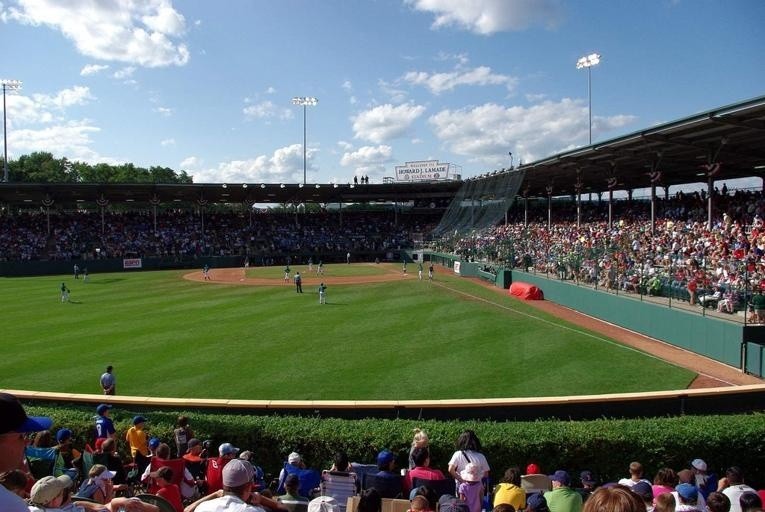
[285, 266, 304, 293]
[1, 203, 422, 265]
[354, 176, 369, 184]
[280, 427, 527, 512]
[402, 259, 434, 279]
[524, 458, 765, 512]
[100, 366, 116, 395]
[1, 392, 289, 512]
[319, 284, 328, 305]
[61, 283, 70, 303]
[308, 257, 324, 276]
[204, 264, 210, 280]
[422, 183, 764, 325]
[74, 264, 87, 280]
[244, 256, 249, 270]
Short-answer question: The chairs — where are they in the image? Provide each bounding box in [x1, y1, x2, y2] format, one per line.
[660, 276, 706, 303]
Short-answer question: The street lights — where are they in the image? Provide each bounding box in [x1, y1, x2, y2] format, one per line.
[0, 78, 24, 183]
[289, 96, 318, 185]
[575, 52, 601, 146]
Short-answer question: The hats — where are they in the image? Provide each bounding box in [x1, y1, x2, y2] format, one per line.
[29, 474, 74, 504]
[0, 390, 52, 436]
[526, 493, 548, 512]
[133, 415, 147, 425]
[149, 437, 161, 449]
[218, 441, 257, 488]
[56, 428, 73, 441]
[460, 462, 485, 482]
[186, 438, 201, 453]
[308, 496, 340, 512]
[627, 482, 654, 501]
[548, 469, 571, 485]
[96, 404, 112, 416]
[376, 450, 395, 468]
[674, 458, 708, 503]
[524, 462, 541, 475]
[578, 470, 594, 484]
[89, 465, 118, 481]
[287, 452, 303, 466]
[438, 493, 470, 512]
